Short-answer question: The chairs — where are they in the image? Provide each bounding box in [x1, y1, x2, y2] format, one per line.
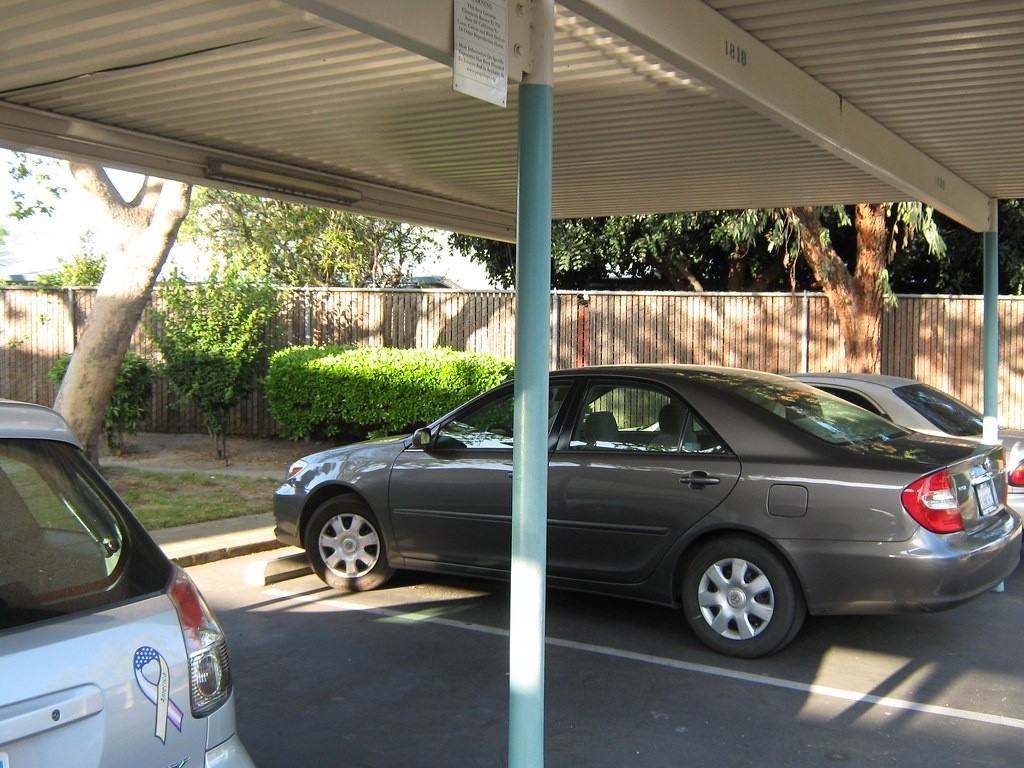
[583, 411, 619, 442]
[649, 404, 697, 447]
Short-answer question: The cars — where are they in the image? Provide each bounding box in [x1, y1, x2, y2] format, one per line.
[645, 373, 1024, 519]
[271, 362, 1024, 659]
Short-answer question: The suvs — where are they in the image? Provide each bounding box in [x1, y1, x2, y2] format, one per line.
[0, 398, 249, 768]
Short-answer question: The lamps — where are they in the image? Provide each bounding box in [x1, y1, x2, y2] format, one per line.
[204, 159, 362, 206]
